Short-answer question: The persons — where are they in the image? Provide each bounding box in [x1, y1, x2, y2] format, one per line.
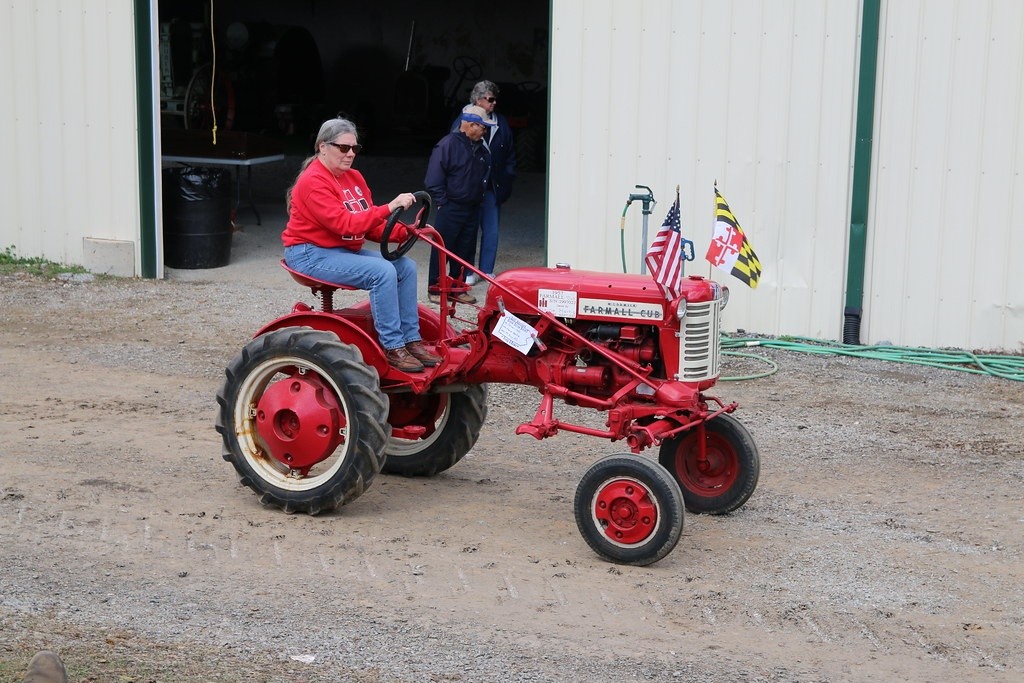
[424, 105, 497, 304]
[281, 118, 444, 369]
[451, 80, 517, 286]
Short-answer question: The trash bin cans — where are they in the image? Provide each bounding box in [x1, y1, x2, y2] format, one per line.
[163, 166, 240, 269]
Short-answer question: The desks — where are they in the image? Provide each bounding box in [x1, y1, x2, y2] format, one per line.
[162, 145, 286, 226]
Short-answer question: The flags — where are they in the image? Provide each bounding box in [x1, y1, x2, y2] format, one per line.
[645, 194, 681, 302]
[705, 187, 762, 290]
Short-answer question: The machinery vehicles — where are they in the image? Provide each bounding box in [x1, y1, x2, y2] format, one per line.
[215, 189, 761, 566]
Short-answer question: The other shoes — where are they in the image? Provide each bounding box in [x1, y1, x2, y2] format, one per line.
[484, 273, 496, 284]
[465, 273, 478, 285]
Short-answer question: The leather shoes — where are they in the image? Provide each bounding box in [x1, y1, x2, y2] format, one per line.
[382, 348, 425, 371]
[428, 293, 440, 304]
[448, 286, 476, 303]
[404, 341, 444, 366]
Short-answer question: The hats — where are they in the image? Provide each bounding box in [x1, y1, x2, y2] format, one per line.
[462, 105, 496, 126]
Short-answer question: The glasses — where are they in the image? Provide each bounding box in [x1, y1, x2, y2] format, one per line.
[329, 142, 363, 155]
[474, 122, 487, 132]
[483, 96, 498, 103]
